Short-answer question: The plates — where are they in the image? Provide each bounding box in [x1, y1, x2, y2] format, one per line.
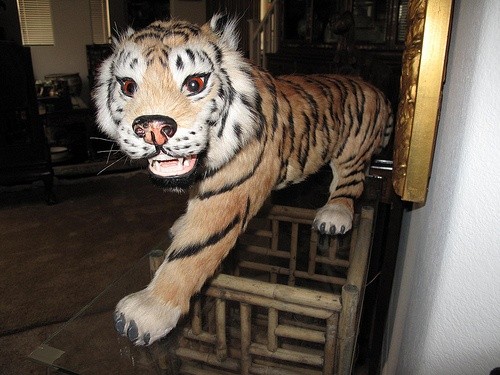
[397, 2, 408, 40]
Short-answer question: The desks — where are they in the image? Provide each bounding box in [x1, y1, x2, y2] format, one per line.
[22, 174, 391, 375]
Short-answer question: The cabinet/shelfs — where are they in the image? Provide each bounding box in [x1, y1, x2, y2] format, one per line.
[259, 1, 406, 106]
[0, 0, 59, 207]
